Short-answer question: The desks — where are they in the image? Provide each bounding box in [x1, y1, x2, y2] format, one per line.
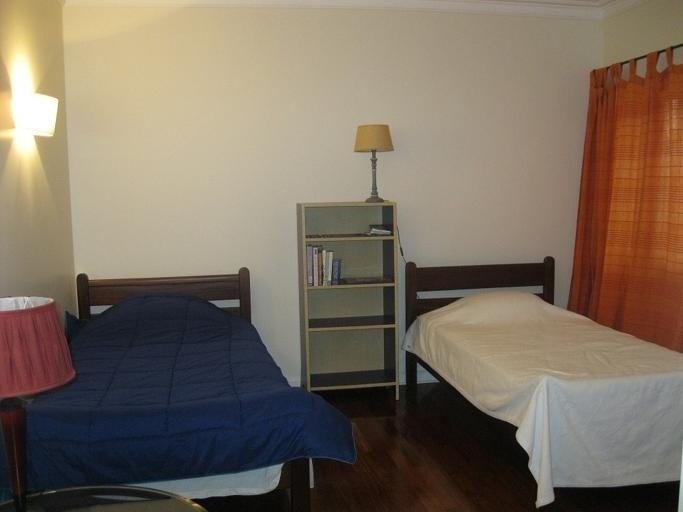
[0, 481, 208, 510]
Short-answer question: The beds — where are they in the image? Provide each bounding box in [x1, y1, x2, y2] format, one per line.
[0, 266, 356, 511]
[403, 254, 682, 506]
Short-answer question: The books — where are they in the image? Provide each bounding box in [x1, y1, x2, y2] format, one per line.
[307, 245, 341, 287]
[368, 224, 392, 236]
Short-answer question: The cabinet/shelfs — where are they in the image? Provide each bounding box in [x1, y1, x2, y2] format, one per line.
[295, 202, 399, 411]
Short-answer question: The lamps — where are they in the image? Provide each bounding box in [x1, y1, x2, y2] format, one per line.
[0, 295, 76, 511]
[14, 93, 60, 139]
[352, 124, 395, 201]
